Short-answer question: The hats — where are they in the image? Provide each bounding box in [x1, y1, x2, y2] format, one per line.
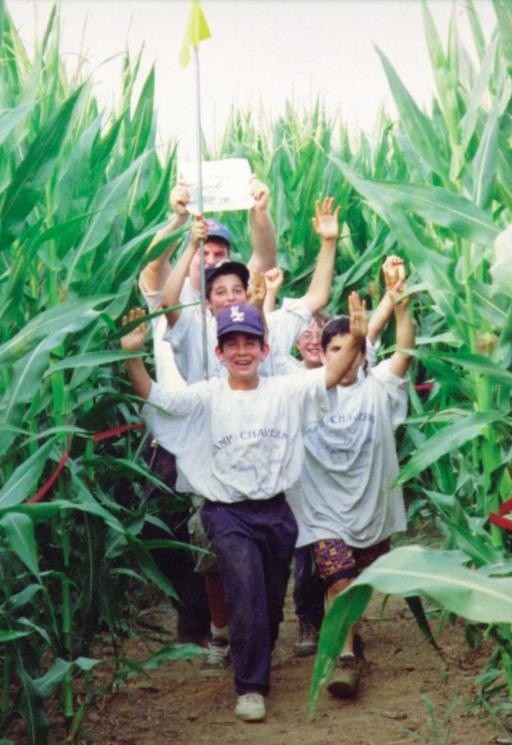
[217, 304, 265, 336]
[205, 261, 248, 282]
[185, 220, 231, 245]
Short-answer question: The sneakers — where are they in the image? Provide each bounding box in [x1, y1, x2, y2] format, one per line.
[328, 636, 365, 697]
[235, 693, 266, 720]
[296, 619, 319, 656]
[199, 638, 230, 677]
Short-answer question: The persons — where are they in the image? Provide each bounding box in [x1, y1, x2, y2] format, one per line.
[121, 175, 416, 722]
